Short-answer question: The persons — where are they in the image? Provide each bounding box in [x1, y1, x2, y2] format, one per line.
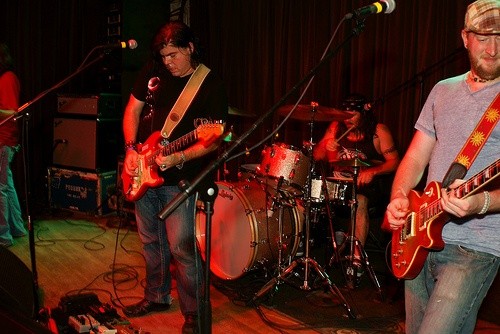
[388, 0, 500, 334]
[0, 44, 26, 247]
[123, 22, 229, 334]
[313, 93, 400, 277]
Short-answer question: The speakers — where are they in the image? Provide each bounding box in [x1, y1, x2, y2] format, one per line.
[0, 244, 55, 334]
[49, 113, 120, 172]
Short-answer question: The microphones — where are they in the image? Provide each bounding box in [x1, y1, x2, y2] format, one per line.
[345, 0, 396, 19]
[56, 139, 68, 143]
[98, 39, 138, 49]
[364, 103, 373, 111]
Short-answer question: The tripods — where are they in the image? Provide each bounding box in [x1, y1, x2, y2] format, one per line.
[245, 110, 385, 320]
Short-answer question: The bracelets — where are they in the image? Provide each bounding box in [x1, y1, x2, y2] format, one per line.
[176, 151, 185, 169]
[125, 143, 136, 152]
[477, 189, 490, 215]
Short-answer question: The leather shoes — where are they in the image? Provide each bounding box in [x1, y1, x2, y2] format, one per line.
[123, 298, 171, 317]
[182, 320, 200, 334]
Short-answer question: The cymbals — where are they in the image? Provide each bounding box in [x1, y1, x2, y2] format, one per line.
[331, 157, 387, 168]
[278, 103, 354, 121]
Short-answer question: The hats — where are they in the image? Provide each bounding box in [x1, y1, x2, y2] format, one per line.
[465, 0, 500, 34]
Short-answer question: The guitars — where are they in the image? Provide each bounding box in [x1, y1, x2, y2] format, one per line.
[390, 158, 500, 281]
[121, 119, 227, 204]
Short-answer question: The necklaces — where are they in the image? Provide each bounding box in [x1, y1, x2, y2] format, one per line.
[469, 72, 488, 82]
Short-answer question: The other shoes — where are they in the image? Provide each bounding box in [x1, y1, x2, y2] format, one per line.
[347, 255, 365, 276]
[0, 240, 13, 247]
[12, 232, 26, 236]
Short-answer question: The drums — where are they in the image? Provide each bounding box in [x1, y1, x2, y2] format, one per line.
[238, 177, 310, 262]
[237, 164, 260, 181]
[259, 142, 311, 187]
[193, 177, 292, 281]
[302, 176, 354, 204]
